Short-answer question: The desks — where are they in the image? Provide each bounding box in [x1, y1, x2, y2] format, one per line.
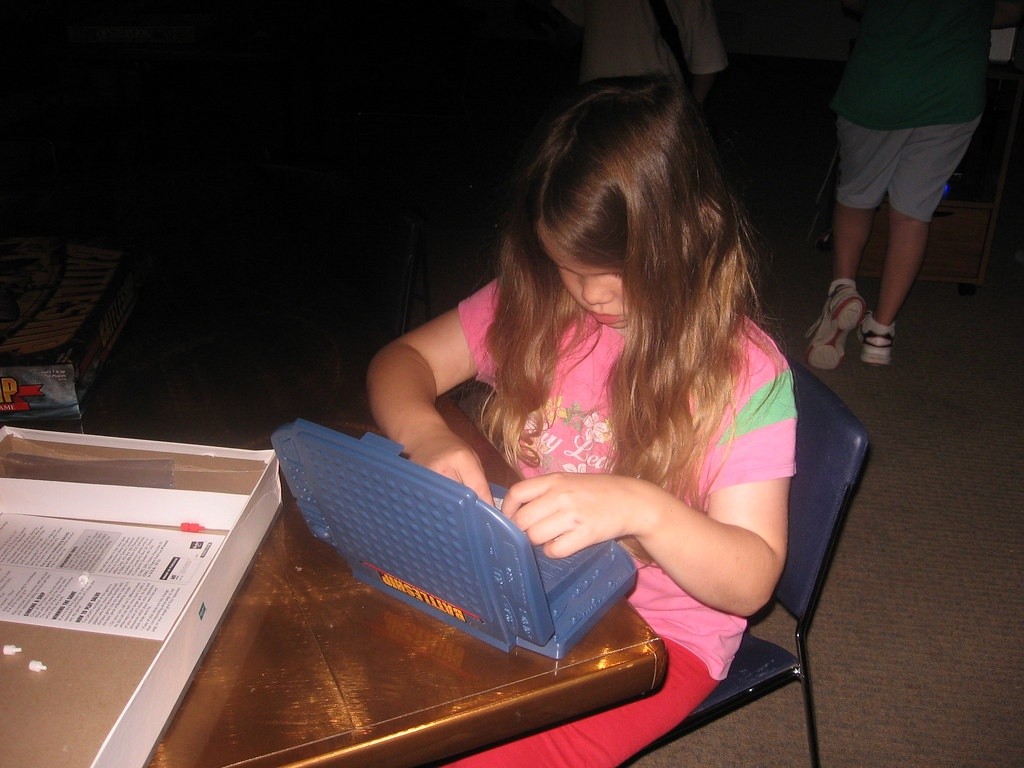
[70, 363, 668, 766]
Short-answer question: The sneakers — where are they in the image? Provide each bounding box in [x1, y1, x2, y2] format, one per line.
[858, 308, 896, 369]
[803, 284, 864, 371]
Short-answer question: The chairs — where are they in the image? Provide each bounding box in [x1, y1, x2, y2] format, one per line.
[619, 343, 873, 766]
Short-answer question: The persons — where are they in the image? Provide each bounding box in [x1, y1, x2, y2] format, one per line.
[537, 0, 734, 139]
[803, 1, 1022, 378]
[350, 67, 800, 768]
